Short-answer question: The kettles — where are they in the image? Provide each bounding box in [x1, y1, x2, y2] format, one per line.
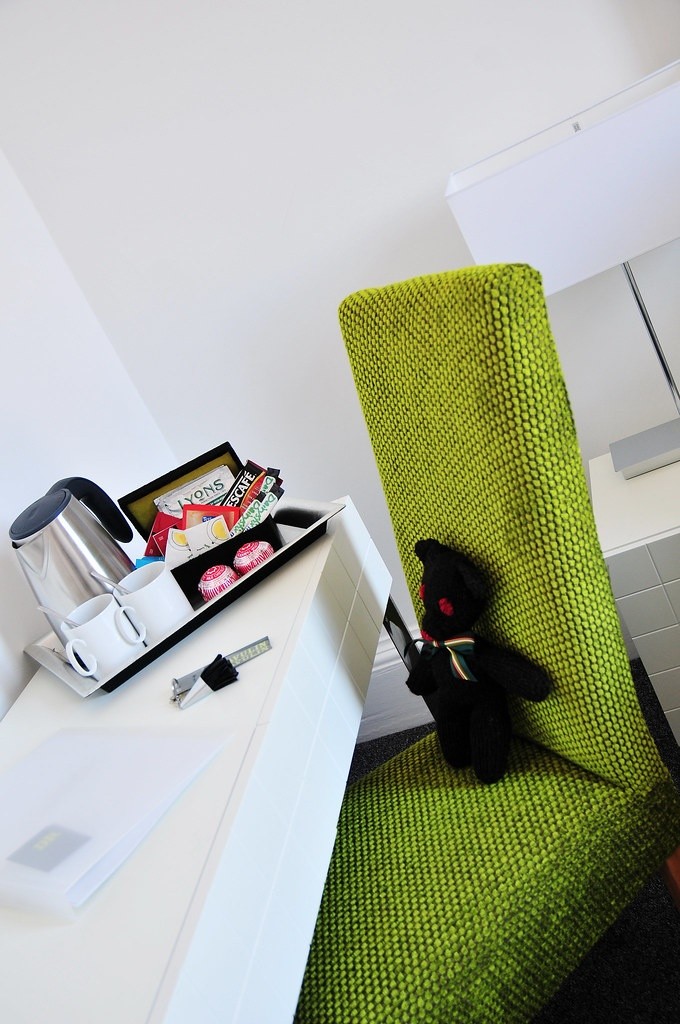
[9, 477, 133, 663]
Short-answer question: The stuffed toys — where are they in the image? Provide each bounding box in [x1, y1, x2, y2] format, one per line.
[406, 538, 554, 785]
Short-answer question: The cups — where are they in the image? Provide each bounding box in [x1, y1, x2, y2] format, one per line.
[60, 593, 145, 682]
[112, 561, 195, 646]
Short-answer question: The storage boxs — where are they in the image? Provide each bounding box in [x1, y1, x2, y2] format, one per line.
[170, 513, 283, 607]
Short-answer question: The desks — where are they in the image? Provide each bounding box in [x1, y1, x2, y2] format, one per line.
[0, 496, 435, 1024]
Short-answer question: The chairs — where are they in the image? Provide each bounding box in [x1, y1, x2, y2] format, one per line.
[290, 262, 680, 1024]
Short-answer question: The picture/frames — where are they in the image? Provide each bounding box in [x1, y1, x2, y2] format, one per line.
[118, 441, 245, 545]
